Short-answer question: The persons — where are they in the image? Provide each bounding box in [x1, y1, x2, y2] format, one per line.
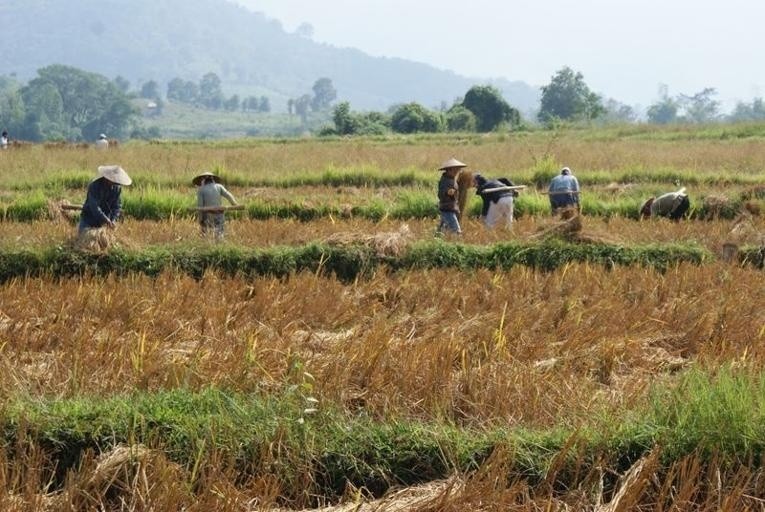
[95, 132, 110, 155]
[192, 171, 239, 241]
[77, 165, 131, 248]
[639, 192, 690, 222]
[437, 157, 520, 237]
[0, 131, 8, 149]
[546, 167, 582, 213]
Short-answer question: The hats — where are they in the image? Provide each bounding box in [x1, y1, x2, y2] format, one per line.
[191, 171, 221, 188]
[438, 157, 468, 172]
[97, 164, 132, 186]
[639, 196, 657, 218]
